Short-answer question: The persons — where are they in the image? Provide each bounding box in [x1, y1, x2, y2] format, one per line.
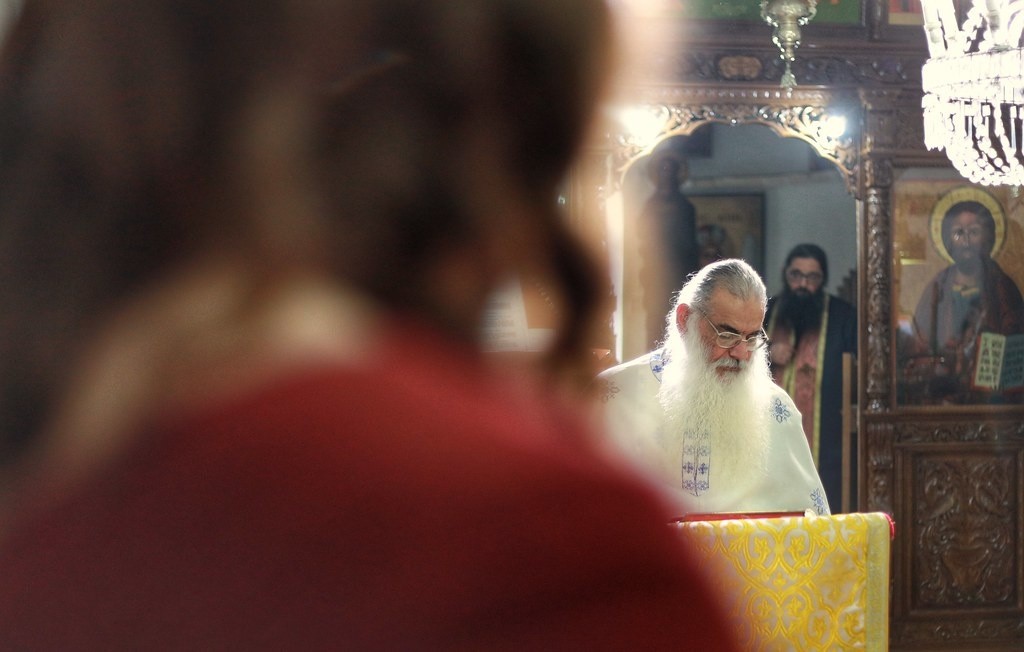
[763, 242, 856, 512]
[0, 0, 740, 651]
[584, 260, 830, 517]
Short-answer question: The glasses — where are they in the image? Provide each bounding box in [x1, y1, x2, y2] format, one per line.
[785, 267, 825, 285]
[691, 304, 769, 353]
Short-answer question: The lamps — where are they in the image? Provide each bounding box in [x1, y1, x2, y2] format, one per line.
[921, 0, 1024, 186]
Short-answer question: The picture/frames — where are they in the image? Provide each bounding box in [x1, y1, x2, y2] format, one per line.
[888, 155, 1024, 412]
[686, 192, 766, 293]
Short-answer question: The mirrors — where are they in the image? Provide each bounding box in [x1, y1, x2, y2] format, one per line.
[621, 102, 864, 515]
